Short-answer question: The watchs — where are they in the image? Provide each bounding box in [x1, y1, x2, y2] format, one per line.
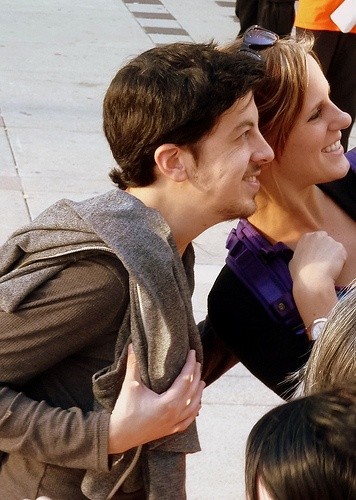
[305, 317, 329, 342]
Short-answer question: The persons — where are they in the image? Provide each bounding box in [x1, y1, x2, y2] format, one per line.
[235, 0, 356, 153]
[0, 35, 275, 500]
[183, 25, 356, 402]
[244, 386, 356, 500]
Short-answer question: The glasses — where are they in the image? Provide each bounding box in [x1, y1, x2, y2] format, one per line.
[231, 26, 279, 61]
